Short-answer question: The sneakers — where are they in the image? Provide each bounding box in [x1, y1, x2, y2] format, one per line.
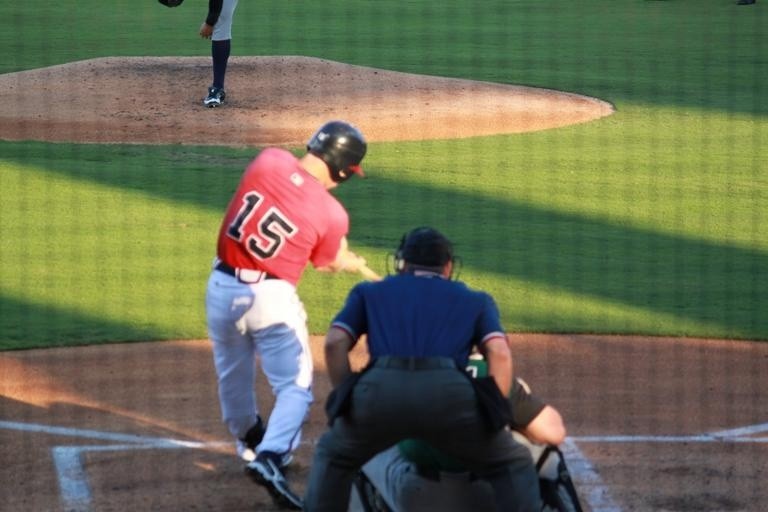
[204, 85, 226, 108]
[239, 412, 305, 511]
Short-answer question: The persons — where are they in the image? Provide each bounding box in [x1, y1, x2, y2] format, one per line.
[159, 1, 235, 107]
[348, 340, 567, 510]
[205, 118, 366, 511]
[301, 226, 542, 511]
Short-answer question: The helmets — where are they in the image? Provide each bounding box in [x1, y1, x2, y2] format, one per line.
[385, 226, 463, 282]
[305, 120, 368, 183]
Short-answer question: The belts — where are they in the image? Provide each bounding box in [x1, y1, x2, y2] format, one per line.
[368, 354, 457, 370]
[214, 261, 279, 280]
